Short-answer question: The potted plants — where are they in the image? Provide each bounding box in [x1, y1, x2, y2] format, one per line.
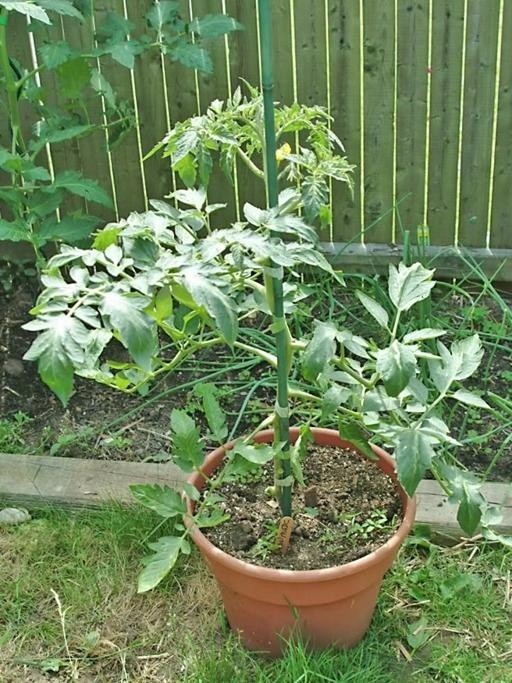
[21, 76, 485, 666]
